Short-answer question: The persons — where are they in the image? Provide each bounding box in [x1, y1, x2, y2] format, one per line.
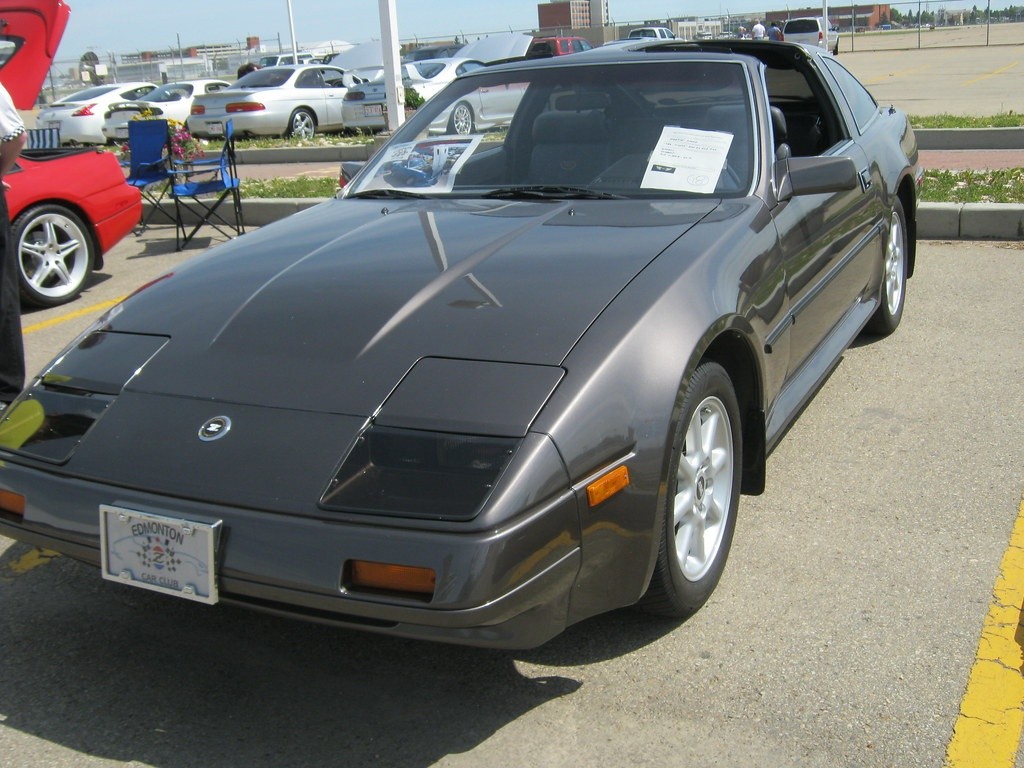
[767, 22, 781, 40]
[752, 19, 765, 40]
[737, 25, 747, 38]
[237, 63, 261, 80]
[0, 80, 27, 415]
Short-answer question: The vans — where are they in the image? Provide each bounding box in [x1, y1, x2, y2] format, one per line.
[628, 27, 682, 42]
[455, 34, 594, 58]
[782, 16, 841, 55]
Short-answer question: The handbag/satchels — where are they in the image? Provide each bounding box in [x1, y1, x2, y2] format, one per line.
[776, 31, 784, 41]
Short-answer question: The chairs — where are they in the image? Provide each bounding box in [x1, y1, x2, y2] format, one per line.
[166, 118, 245, 253]
[26, 128, 61, 150]
[705, 105, 788, 192]
[520, 108, 615, 189]
[555, 93, 614, 116]
[120, 118, 186, 241]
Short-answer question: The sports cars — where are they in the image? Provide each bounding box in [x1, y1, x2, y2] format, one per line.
[0, 37, 922, 654]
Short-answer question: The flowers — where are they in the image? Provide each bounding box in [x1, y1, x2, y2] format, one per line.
[121, 106, 205, 165]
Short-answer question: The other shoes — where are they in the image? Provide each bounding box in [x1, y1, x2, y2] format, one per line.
[0, 401, 8, 410]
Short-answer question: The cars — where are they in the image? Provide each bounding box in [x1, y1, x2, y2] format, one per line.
[101, 78, 233, 141]
[188, 64, 371, 140]
[259, 37, 466, 93]
[0, 0, 143, 309]
[37, 80, 179, 145]
[340, 58, 489, 131]
